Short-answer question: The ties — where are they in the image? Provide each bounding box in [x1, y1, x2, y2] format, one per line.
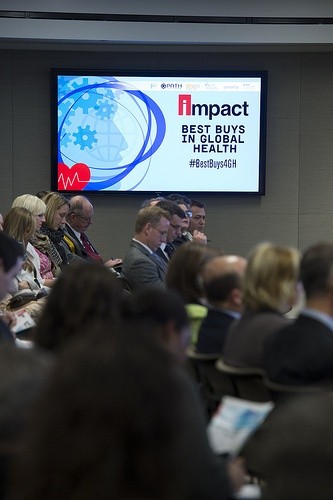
[80, 234, 103, 265]
[63, 235, 75, 254]
[164, 245, 174, 261]
[152, 251, 167, 271]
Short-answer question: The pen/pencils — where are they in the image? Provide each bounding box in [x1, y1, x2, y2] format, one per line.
[190, 237, 212, 242]
[112, 257, 113, 260]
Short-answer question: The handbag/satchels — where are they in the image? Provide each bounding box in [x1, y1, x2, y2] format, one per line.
[6, 292, 37, 309]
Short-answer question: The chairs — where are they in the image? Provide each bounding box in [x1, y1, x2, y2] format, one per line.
[188, 352, 328, 483]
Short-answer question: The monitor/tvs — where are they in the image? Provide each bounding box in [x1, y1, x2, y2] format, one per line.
[49, 68, 267, 197]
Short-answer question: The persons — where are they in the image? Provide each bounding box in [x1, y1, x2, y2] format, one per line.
[0, 192, 333, 500]
[121, 206, 170, 292]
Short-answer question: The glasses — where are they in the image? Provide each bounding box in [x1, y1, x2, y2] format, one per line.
[185, 210, 193, 218]
